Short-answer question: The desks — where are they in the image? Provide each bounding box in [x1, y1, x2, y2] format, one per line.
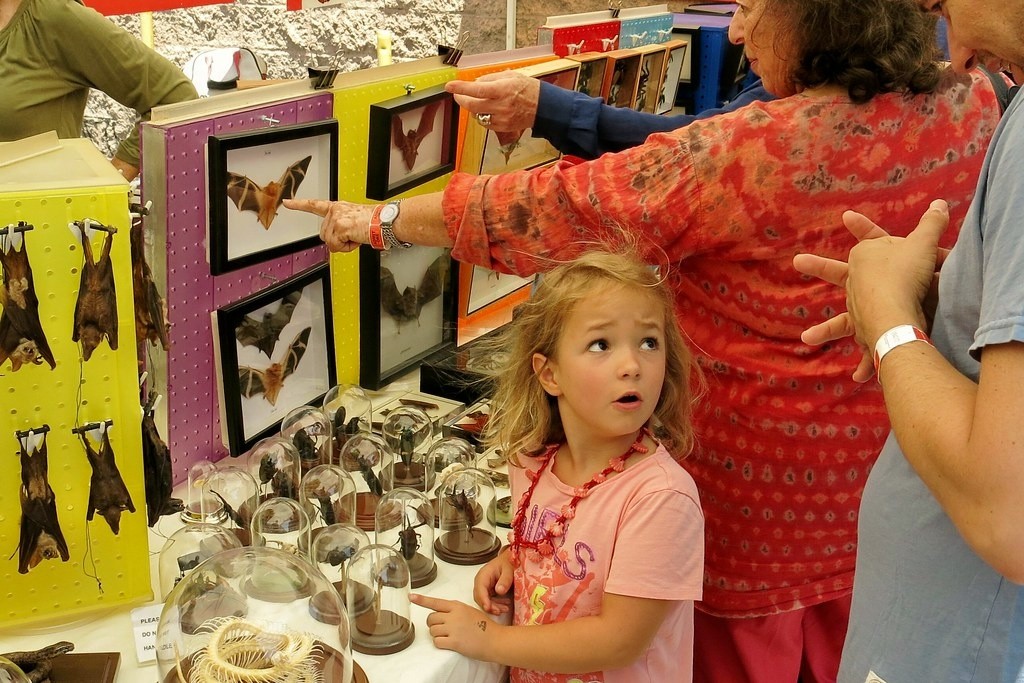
[0, 368, 514, 682]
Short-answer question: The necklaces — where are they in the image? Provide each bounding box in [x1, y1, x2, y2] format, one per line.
[507, 426, 649, 569]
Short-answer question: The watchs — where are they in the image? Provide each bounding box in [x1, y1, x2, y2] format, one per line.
[380, 198, 413, 250]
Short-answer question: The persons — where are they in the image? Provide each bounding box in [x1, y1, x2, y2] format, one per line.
[409, 251, 705, 683]
[793, 0, 1024, 683]
[282, 0, 1000, 683]
[0, 0, 199, 183]
[444, 17, 949, 162]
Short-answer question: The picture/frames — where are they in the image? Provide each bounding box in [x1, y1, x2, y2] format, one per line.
[208, 118, 338, 277]
[458, 39, 687, 176]
[358, 244, 537, 406]
[217, 259, 338, 459]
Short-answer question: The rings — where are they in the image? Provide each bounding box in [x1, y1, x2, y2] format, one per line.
[476, 114, 492, 126]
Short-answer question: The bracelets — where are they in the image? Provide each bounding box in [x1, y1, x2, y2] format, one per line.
[369, 204, 392, 251]
[874, 324, 934, 385]
